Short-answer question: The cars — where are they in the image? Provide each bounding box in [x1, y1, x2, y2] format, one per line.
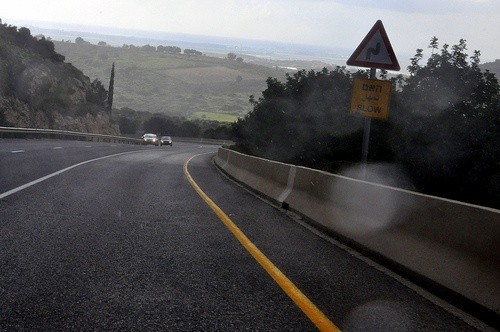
[141, 133, 159, 146]
[160, 136, 172, 147]
